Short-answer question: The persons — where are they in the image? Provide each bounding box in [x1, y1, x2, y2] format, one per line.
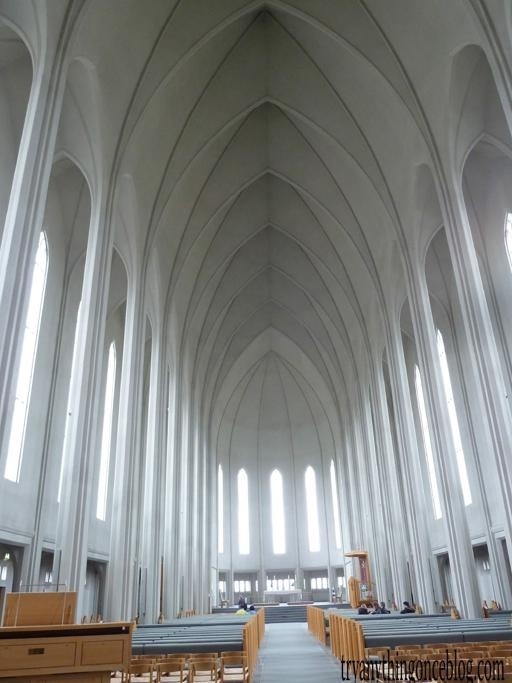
[236, 594, 256, 615]
[358, 601, 415, 615]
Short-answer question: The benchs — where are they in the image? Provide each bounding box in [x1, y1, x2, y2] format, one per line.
[305, 605, 512, 670]
[131, 608, 265, 676]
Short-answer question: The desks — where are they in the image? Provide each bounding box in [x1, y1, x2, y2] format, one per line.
[0, 621, 134, 683]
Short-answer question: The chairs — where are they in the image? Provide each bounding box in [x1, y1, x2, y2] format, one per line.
[365, 641, 512, 683]
[121, 650, 248, 683]
